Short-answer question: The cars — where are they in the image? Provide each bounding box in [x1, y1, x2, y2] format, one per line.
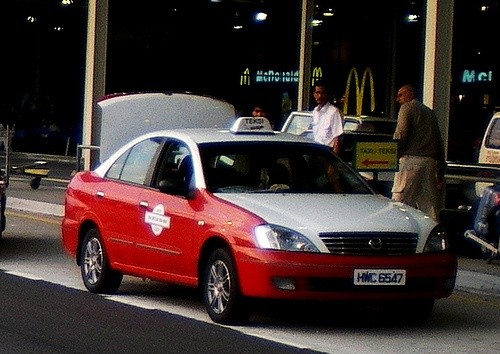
[279, 102, 500, 266]
[59, 115, 458, 326]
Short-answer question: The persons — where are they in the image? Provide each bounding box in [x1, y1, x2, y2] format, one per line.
[251, 100, 266, 117]
[391, 85, 446, 221]
[308, 80, 343, 174]
